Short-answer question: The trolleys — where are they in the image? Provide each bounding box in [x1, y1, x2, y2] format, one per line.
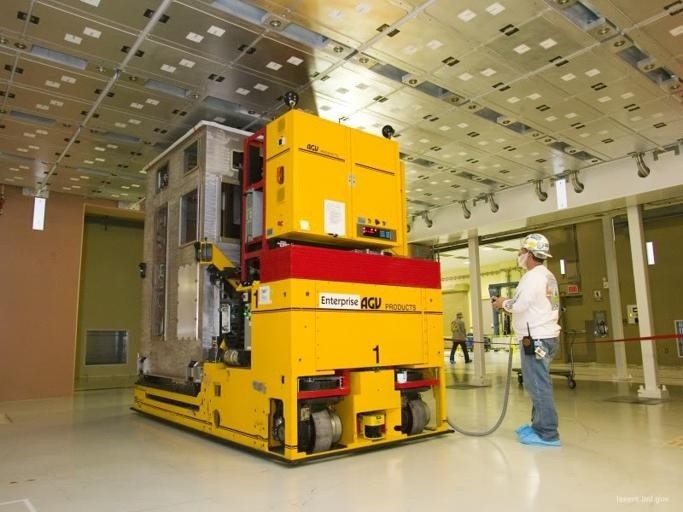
[512, 329, 577, 389]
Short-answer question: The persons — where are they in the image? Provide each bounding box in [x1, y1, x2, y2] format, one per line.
[448, 312, 472, 364]
[488, 232, 564, 448]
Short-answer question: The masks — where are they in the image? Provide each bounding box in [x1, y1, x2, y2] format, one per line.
[518, 251, 529, 269]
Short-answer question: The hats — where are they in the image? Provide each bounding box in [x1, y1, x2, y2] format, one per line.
[457, 312, 463, 317]
[522, 233, 553, 259]
